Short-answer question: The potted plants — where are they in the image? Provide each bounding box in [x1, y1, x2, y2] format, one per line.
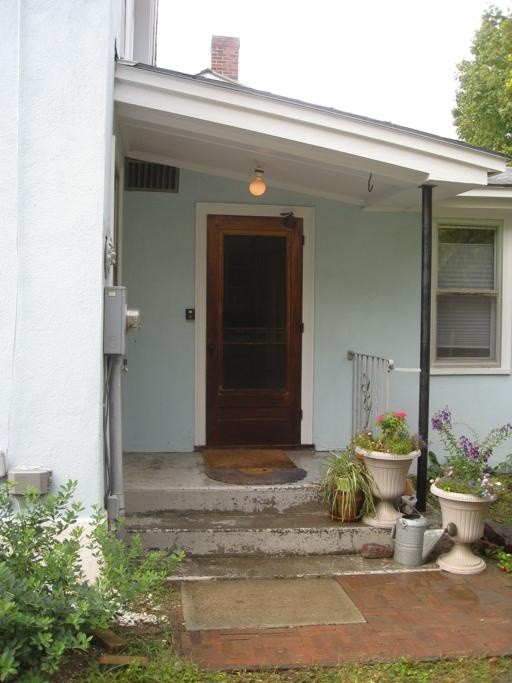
[321, 449, 377, 522]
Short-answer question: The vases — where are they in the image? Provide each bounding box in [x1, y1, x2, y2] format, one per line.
[428, 482, 492, 576]
[355, 446, 420, 528]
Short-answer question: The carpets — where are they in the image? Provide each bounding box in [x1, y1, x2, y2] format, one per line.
[200, 443, 307, 488]
[181, 572, 370, 633]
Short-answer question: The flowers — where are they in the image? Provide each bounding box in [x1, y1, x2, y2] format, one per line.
[347, 407, 423, 455]
[421, 405, 512, 501]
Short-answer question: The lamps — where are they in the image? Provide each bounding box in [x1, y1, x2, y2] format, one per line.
[249, 157, 266, 197]
